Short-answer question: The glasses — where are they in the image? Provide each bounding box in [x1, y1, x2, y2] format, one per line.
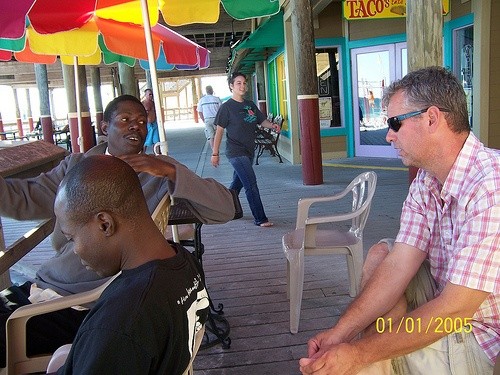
[387, 108, 451, 132]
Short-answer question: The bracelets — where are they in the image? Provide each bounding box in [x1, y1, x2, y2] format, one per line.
[272, 123, 277, 127]
[212, 154, 217, 156]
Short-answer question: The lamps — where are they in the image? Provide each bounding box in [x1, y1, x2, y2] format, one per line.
[225, 19, 240, 74]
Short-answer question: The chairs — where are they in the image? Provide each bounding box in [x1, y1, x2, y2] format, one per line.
[283, 171, 377, 334]
[5, 191, 173, 375]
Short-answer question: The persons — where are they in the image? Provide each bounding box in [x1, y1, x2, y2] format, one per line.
[0, 72, 282, 375]
[300, 67, 500, 375]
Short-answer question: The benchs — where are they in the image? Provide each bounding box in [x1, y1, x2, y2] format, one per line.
[253, 113, 285, 165]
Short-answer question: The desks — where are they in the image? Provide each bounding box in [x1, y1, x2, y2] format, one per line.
[166, 188, 243, 351]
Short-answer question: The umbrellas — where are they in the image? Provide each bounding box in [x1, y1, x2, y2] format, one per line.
[0, 0, 286, 250]
